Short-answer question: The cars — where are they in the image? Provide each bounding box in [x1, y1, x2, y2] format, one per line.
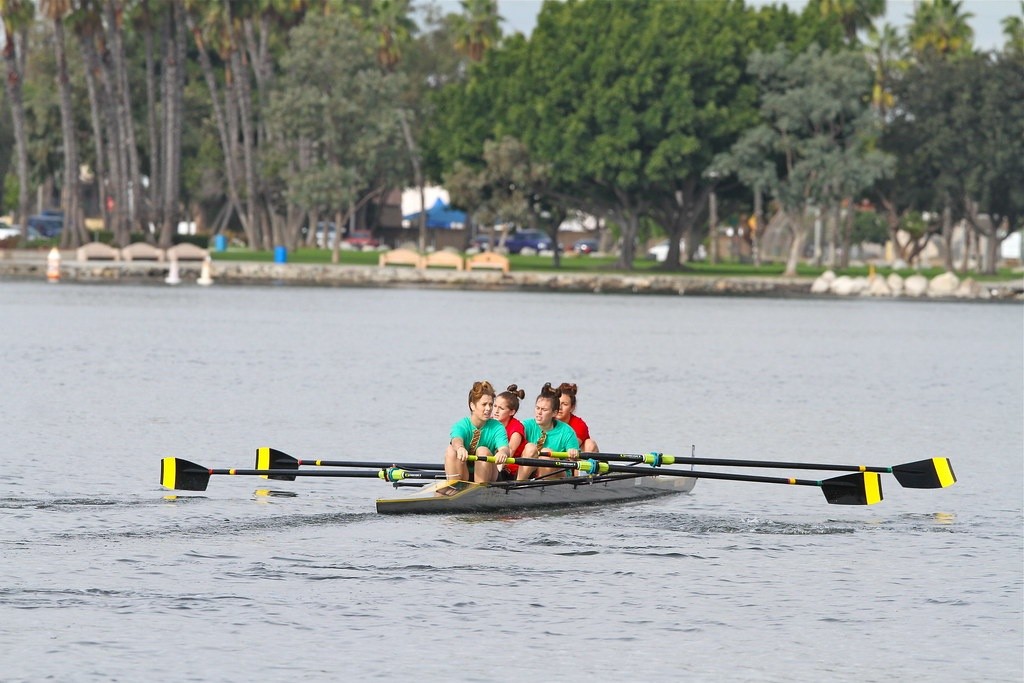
[572, 236, 600, 255]
[341, 229, 381, 247]
[645, 237, 707, 262]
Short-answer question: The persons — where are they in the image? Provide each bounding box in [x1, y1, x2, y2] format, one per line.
[444, 381, 600, 486]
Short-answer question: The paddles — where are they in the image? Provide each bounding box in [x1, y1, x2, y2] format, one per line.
[542, 451, 957, 490]
[256, 446, 444, 482]
[160, 456, 444, 490]
[466, 453, 883, 506]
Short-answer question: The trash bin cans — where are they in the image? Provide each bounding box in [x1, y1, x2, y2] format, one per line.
[215, 234, 226, 252]
[274, 246, 288, 265]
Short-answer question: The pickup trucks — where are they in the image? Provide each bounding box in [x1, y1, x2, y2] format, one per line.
[468, 227, 552, 255]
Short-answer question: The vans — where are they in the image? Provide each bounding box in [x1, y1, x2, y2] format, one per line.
[26, 216, 99, 245]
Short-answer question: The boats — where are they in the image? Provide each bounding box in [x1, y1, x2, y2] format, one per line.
[375, 475, 699, 515]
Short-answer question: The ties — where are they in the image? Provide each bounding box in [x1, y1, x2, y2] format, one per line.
[537, 431, 547, 456]
[468, 429, 480, 467]
